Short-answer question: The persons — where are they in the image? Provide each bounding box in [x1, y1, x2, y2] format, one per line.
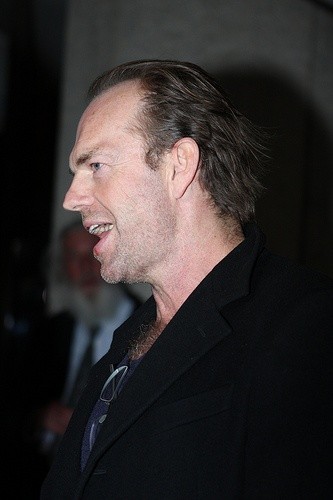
[10, 220, 144, 500]
[37, 58, 333, 499]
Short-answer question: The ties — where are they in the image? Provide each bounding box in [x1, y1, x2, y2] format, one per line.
[80, 363, 129, 474]
[67, 325, 102, 408]
